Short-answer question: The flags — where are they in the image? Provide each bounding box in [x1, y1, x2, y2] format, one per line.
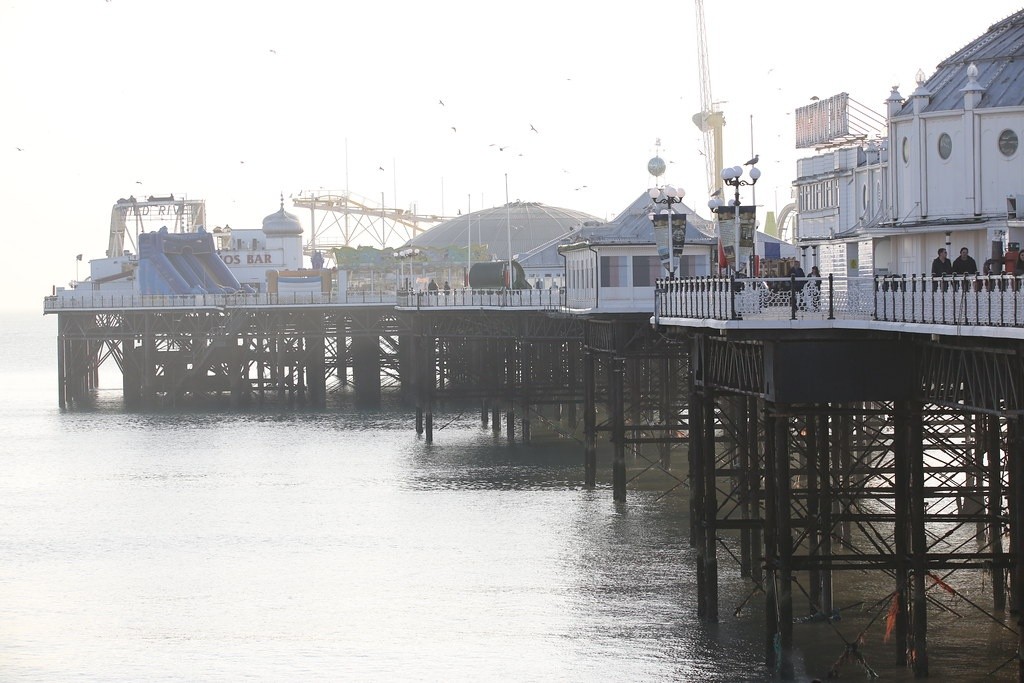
[719, 240, 728, 268]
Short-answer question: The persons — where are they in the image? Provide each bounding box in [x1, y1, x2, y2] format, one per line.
[443, 281, 450, 295]
[764, 271, 777, 293]
[1012, 251, 1024, 276]
[953, 247, 977, 274]
[428, 279, 438, 295]
[536, 278, 543, 289]
[786, 261, 806, 309]
[932, 248, 951, 274]
[807, 266, 822, 312]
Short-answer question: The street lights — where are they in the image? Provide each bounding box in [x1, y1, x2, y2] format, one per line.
[394, 250, 408, 290]
[650, 187, 685, 281]
[721, 166, 760, 320]
[404, 249, 419, 291]
[648, 209, 675, 277]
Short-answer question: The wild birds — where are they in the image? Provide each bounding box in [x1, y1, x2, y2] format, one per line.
[16, 47, 792, 223]
[810, 96, 820, 101]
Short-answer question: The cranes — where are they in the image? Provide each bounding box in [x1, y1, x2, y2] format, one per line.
[691, 1, 725, 223]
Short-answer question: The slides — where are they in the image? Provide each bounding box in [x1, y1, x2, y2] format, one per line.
[157, 252, 256, 299]
